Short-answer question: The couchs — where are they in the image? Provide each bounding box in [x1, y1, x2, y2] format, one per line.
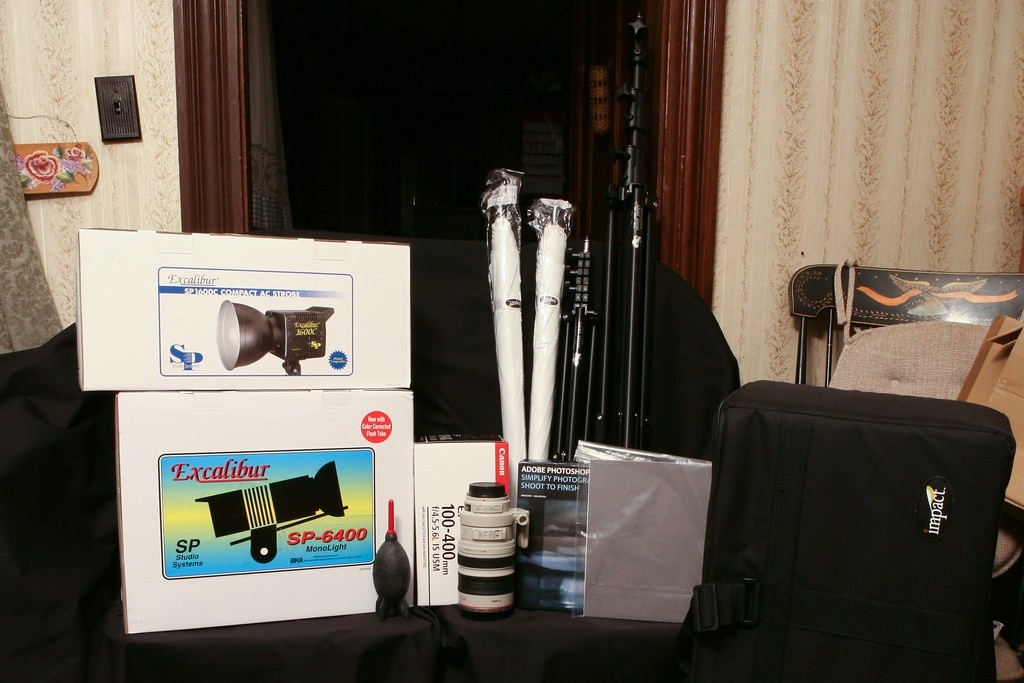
[0, 230, 740, 683]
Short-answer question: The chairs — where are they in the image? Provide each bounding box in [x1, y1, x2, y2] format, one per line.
[790, 264, 1024, 388]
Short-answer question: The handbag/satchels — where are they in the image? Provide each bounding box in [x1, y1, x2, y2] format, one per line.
[690, 380, 1017, 683]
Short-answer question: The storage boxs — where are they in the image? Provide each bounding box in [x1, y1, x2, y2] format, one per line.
[413, 433, 511, 609]
[511, 461, 589, 613]
[115, 391, 414, 635]
[955, 316, 1024, 508]
[76, 229, 410, 393]
